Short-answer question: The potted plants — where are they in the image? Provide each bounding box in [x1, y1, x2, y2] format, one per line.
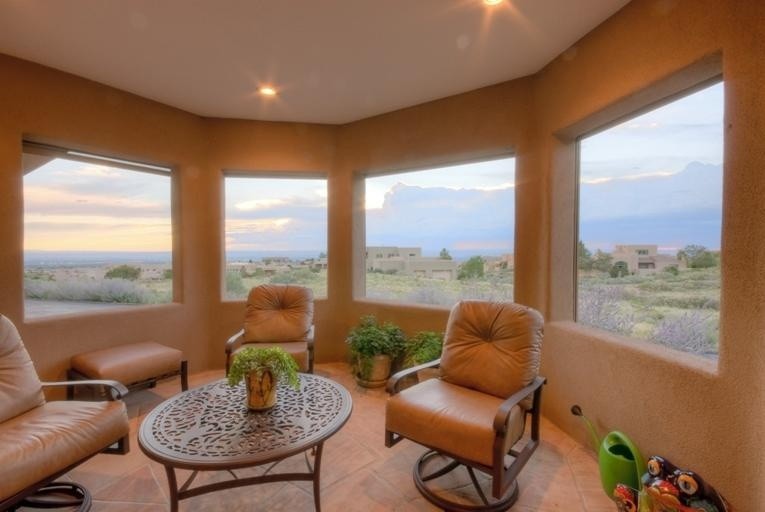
[227, 346, 300, 411]
[402, 331, 446, 383]
[345, 312, 409, 388]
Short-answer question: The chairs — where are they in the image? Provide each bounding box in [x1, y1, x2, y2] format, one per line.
[224, 283, 316, 378]
[383, 299, 549, 512]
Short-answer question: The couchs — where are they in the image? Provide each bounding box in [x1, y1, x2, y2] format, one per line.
[0, 314, 131, 512]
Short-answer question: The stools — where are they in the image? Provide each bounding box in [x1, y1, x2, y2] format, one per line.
[66, 340, 188, 401]
